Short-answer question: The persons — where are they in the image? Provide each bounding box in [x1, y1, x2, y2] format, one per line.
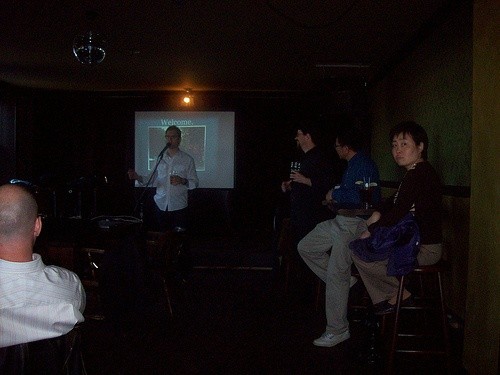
[351, 123, 444, 317]
[281, 124, 333, 243]
[0, 184, 87, 349]
[127, 126, 198, 233]
[296, 131, 380, 347]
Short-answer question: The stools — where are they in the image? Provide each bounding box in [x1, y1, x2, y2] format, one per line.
[378, 266, 453, 374]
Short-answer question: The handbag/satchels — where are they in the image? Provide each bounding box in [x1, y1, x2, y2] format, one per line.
[367, 220, 415, 253]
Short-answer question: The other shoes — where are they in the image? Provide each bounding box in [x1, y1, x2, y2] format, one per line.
[375, 294, 415, 316]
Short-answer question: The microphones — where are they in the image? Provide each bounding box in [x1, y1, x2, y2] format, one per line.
[159, 143, 170, 155]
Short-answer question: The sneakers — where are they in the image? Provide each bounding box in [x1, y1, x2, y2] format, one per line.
[349, 276, 357, 287]
[313, 330, 351, 347]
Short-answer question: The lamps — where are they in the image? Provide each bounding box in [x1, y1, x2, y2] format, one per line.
[71, 10, 108, 65]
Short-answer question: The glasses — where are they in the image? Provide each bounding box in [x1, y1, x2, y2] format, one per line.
[165, 135, 178, 139]
